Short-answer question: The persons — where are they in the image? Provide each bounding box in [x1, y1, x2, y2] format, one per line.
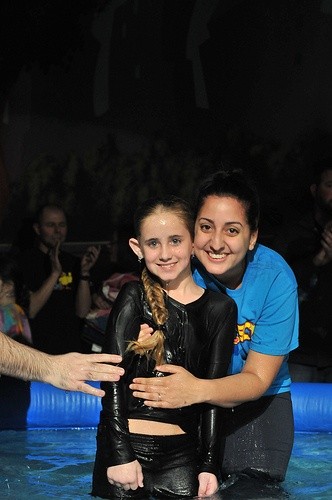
[273, 161, 332, 384]
[89, 197, 238, 500]
[128, 175, 299, 500]
[0, 202, 147, 399]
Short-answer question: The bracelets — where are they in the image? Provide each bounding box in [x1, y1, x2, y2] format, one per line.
[79, 273, 91, 280]
